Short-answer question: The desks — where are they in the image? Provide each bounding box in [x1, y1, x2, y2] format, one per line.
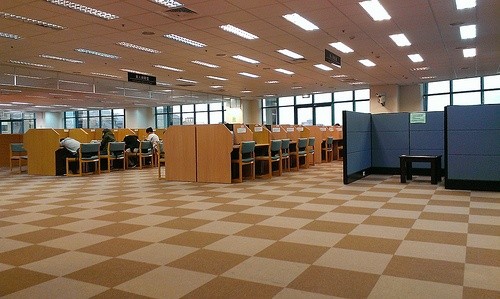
[0, 124, 344, 184]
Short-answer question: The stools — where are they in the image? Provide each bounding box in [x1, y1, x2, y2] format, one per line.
[399, 155, 441, 185]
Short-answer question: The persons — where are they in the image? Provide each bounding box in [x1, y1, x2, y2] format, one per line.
[100, 129, 120, 156]
[144, 127, 165, 150]
[123, 135, 141, 152]
[60, 137, 82, 176]
